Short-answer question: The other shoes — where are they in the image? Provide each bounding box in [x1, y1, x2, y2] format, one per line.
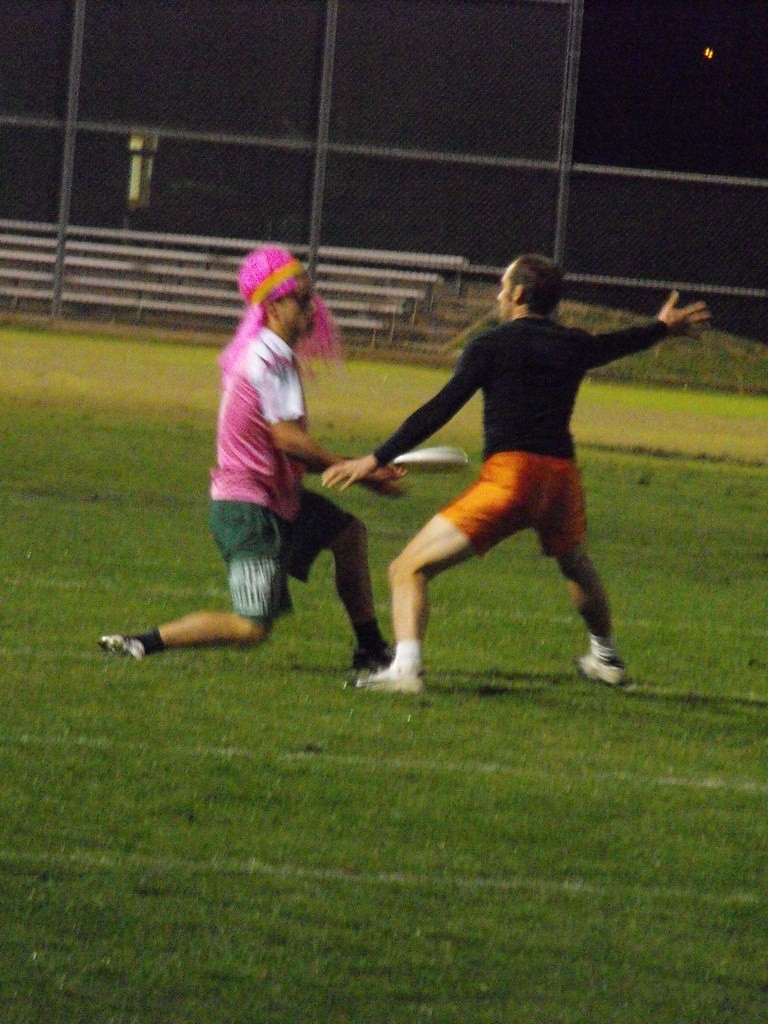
[354, 653, 396, 669]
[98, 633, 147, 662]
[574, 652, 627, 687]
[355, 670, 428, 698]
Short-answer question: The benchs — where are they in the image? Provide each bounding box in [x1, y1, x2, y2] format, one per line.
[1, 217, 467, 350]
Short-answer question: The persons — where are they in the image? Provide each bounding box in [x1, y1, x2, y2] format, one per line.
[321, 254, 714, 695]
[97, 245, 407, 670]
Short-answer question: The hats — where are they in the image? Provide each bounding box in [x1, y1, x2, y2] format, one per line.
[235, 244, 313, 309]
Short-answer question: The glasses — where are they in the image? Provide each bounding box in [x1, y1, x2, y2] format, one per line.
[279, 291, 313, 307]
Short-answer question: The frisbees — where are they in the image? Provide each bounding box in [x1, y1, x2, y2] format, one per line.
[393, 445, 467, 474]
